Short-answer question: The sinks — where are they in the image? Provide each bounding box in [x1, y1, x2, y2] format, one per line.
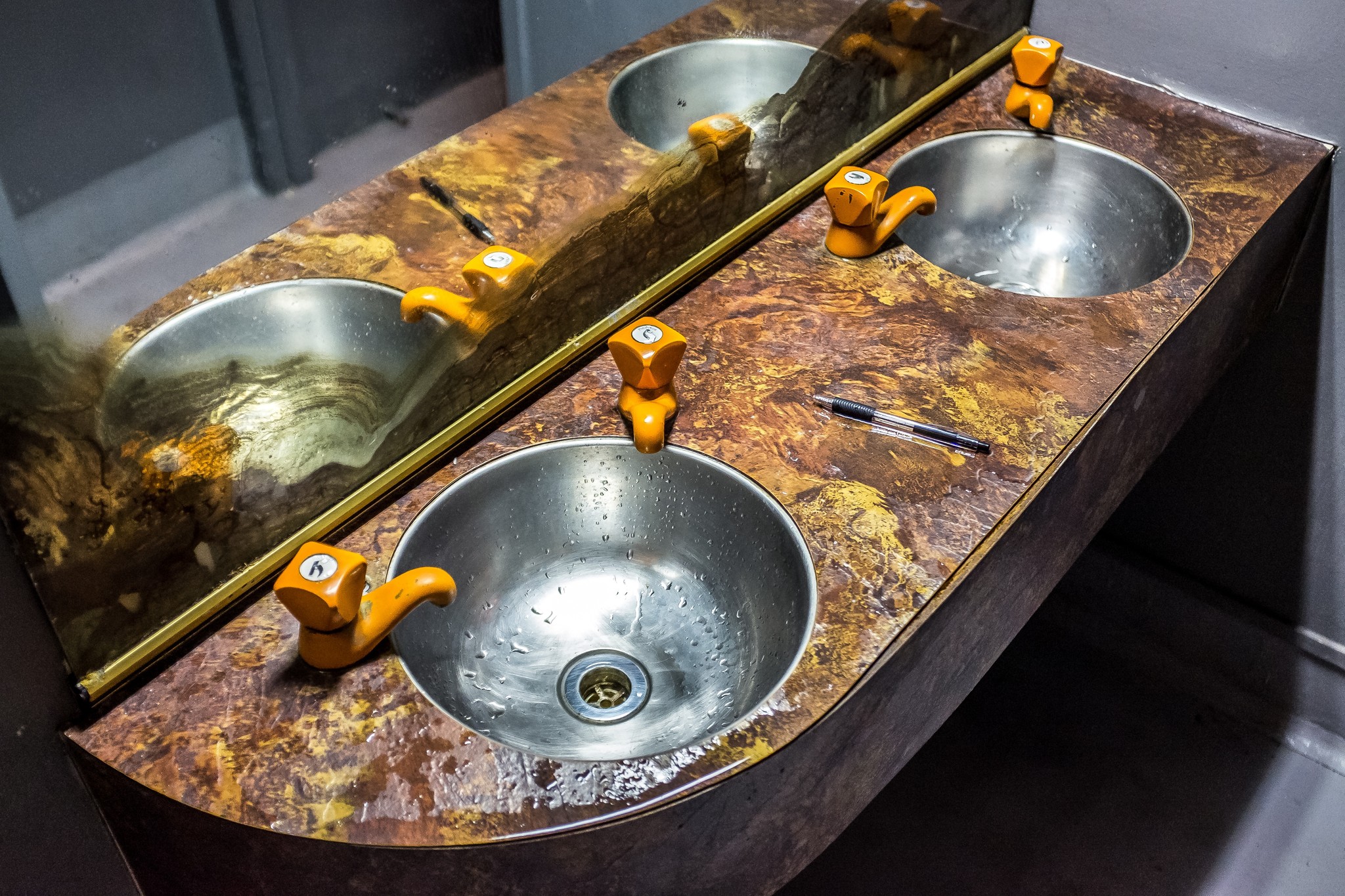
[882, 129, 1194, 297]
[101, 277, 458, 512]
[608, 37, 877, 166]
[386, 437, 818, 761]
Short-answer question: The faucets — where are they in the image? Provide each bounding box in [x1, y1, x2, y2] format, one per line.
[607, 316, 687, 452]
[122, 422, 237, 512]
[1002, 36, 1065, 132]
[821, 164, 941, 256]
[845, 0, 942, 105]
[397, 243, 540, 367]
[684, 112, 774, 224]
[273, 539, 458, 667]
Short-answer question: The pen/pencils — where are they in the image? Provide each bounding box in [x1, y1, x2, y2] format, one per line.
[417, 174, 500, 248]
[808, 394, 992, 455]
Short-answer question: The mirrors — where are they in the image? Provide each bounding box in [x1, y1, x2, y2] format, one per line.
[0, 1, 1032, 707]
